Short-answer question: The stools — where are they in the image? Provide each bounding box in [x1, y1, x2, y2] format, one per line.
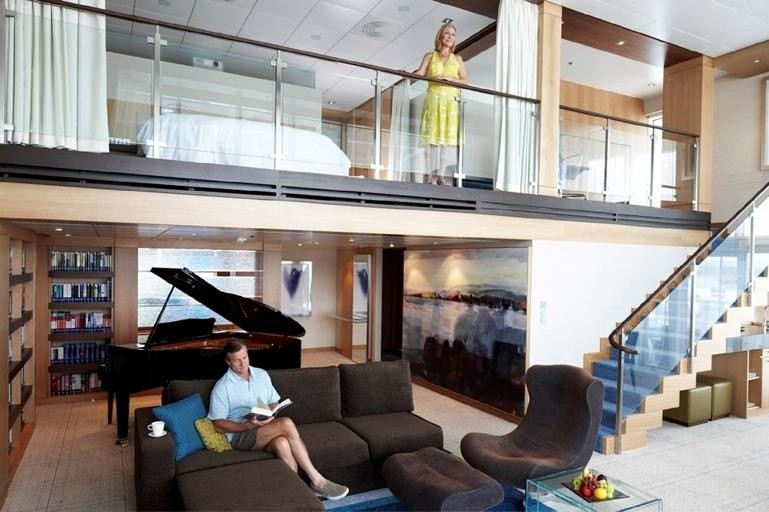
[383, 445, 507, 510]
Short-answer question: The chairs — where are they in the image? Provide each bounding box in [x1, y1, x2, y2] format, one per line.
[460, 364, 604, 485]
[698, 372, 733, 423]
[558, 150, 592, 201]
[663, 381, 712, 428]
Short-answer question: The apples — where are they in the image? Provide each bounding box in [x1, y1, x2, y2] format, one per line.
[580, 485, 593, 497]
[572, 477, 582, 487]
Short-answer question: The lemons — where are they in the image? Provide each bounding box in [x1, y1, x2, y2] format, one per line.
[593, 488, 608, 499]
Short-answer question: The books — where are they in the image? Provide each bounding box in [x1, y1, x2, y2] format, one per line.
[48, 369, 105, 396]
[48, 247, 113, 273]
[50, 338, 111, 363]
[238, 397, 292, 421]
[49, 309, 111, 333]
[50, 278, 112, 303]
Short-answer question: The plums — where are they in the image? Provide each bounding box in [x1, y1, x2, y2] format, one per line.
[597, 474, 607, 481]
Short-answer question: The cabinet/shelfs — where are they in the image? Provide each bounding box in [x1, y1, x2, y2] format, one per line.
[0, 228, 35, 511]
[711, 348, 769, 419]
[50, 233, 115, 405]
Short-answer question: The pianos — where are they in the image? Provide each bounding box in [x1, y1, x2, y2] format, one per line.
[107, 268, 305, 437]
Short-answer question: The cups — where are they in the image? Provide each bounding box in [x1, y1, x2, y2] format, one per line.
[148, 421, 164, 435]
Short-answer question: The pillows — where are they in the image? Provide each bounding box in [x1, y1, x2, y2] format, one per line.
[151, 393, 215, 463]
[191, 414, 232, 452]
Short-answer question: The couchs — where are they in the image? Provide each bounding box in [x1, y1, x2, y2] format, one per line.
[133, 359, 445, 511]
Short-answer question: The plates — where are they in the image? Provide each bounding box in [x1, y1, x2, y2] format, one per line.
[147, 431, 167, 438]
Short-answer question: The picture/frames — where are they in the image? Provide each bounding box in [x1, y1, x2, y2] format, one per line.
[398, 238, 533, 425]
[281, 259, 312, 318]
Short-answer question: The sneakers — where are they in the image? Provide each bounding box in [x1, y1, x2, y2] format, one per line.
[311, 480, 349, 501]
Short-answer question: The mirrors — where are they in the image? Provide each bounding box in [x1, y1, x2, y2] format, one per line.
[351, 253, 372, 364]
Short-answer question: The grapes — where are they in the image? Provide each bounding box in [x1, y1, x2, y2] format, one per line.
[583, 474, 599, 491]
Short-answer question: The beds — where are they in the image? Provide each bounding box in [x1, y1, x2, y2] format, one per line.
[137, 113, 353, 175]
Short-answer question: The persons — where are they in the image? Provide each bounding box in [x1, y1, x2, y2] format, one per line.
[206, 337, 350, 501]
[399, 24, 468, 185]
